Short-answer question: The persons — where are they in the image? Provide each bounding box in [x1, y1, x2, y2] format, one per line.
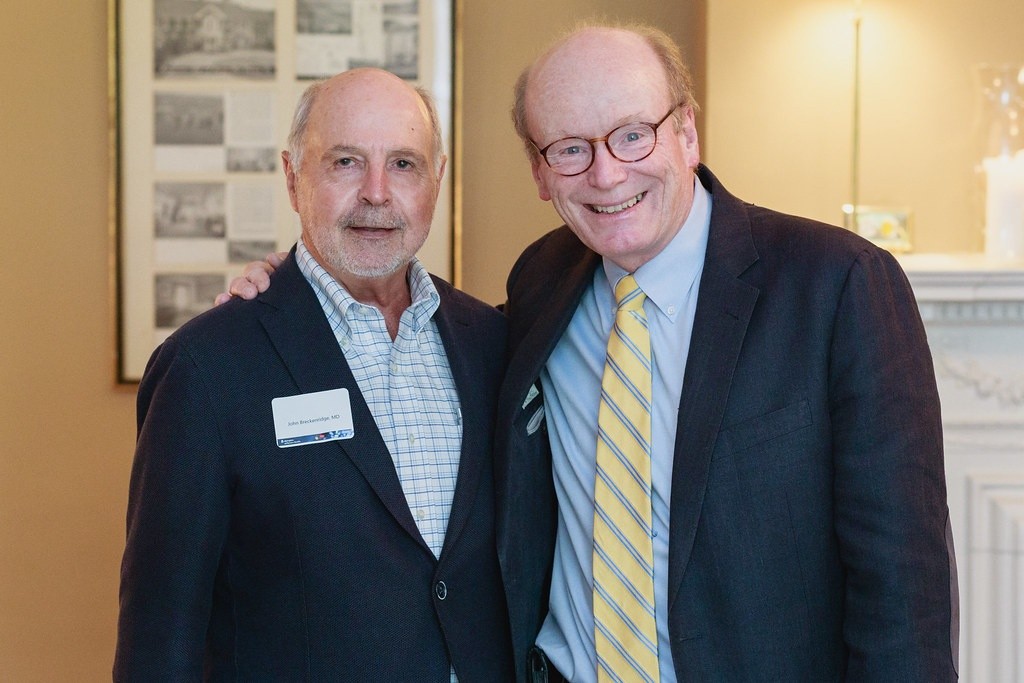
[107, 65, 518, 683]
[215, 21, 966, 683]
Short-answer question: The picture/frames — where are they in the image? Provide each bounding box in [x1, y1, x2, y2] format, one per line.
[107, 0, 463, 394]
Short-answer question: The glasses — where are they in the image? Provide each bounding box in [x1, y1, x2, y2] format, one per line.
[528, 110, 674, 176]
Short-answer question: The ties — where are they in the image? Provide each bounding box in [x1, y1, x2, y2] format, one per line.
[592, 274, 660, 683]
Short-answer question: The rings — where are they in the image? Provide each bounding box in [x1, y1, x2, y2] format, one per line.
[228, 288, 233, 296]
